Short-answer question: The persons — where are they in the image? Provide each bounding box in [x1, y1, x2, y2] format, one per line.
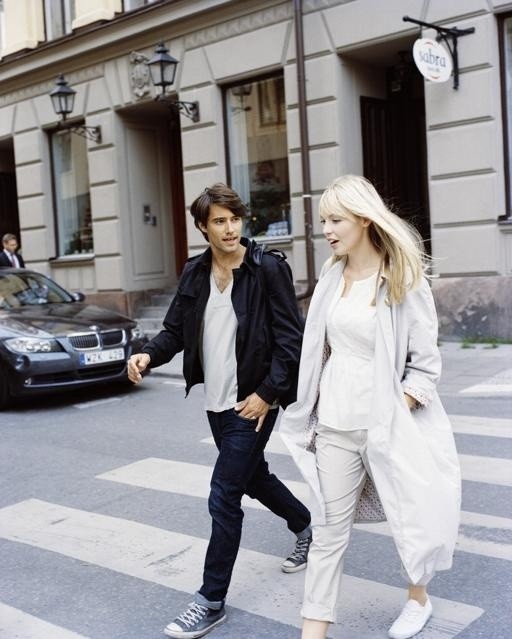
[279, 176, 463, 639]
[251, 161, 281, 234]
[0, 234, 25, 269]
[126, 181, 312, 639]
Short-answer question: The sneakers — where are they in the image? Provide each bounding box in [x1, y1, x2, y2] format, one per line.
[163, 602, 227, 639]
[387, 593, 432, 639]
[281, 535, 312, 573]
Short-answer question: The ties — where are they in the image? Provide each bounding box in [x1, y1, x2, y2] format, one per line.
[11, 254, 16, 267]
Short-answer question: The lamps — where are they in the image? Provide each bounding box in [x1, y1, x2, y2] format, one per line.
[50, 71, 103, 144]
[144, 40, 201, 124]
[231, 84, 254, 113]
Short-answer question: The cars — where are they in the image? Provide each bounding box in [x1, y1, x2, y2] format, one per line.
[0, 266, 150, 410]
